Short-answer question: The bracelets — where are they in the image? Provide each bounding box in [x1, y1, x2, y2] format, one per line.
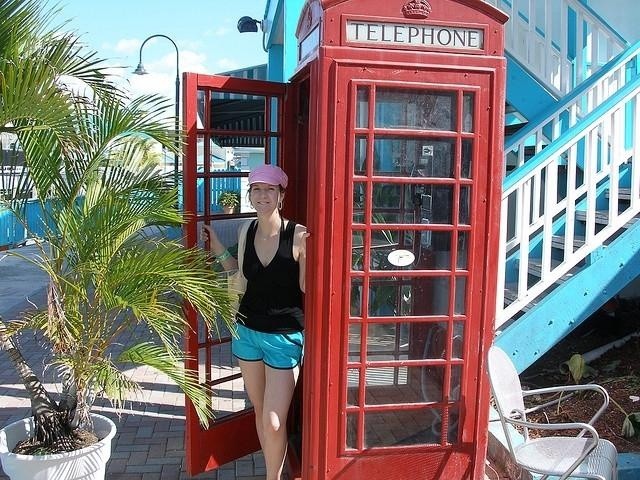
[216, 248, 230, 264]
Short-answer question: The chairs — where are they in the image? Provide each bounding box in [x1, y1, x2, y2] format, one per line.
[485, 342, 620, 480]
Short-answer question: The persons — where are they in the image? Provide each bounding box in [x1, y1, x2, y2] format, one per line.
[200, 163, 312, 480]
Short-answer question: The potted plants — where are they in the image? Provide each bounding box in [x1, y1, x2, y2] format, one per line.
[216, 190, 239, 215]
[0, 0, 247, 480]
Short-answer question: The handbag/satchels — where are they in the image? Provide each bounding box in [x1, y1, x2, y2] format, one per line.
[227, 219, 254, 318]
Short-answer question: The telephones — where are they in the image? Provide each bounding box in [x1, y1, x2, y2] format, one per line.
[413, 140, 471, 251]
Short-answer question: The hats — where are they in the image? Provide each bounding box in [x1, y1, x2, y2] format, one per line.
[247, 164, 289, 190]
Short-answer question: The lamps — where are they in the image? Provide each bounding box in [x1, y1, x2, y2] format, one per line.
[236, 13, 269, 53]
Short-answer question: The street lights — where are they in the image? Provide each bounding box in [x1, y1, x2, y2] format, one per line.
[131, 33, 180, 229]
